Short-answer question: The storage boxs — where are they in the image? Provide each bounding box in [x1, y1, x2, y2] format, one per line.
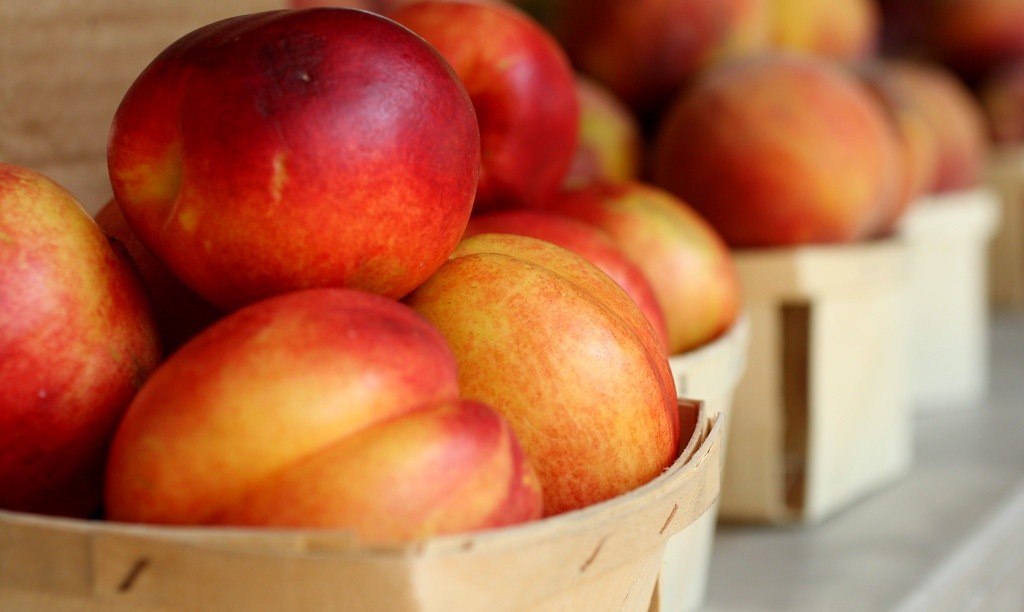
[702, 238, 909, 521]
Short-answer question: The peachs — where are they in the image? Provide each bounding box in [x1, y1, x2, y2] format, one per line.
[0, 0, 1024, 540]
